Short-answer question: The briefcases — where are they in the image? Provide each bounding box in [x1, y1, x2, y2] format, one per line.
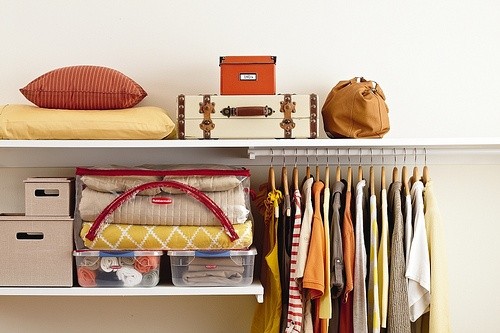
[177, 95, 316, 138]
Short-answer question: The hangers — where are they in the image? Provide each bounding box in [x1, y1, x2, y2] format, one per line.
[266, 148, 429, 218]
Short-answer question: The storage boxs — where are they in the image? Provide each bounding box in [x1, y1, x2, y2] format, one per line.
[219, 55, 277, 96]
[72, 249, 163, 287]
[23, 176, 74, 217]
[0, 212, 75, 288]
[168, 248, 257, 287]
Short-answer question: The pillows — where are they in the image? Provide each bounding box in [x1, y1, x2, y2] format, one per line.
[19, 65, 147, 110]
[0, 104, 177, 141]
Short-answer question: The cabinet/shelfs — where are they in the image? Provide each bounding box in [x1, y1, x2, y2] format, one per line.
[0, 139, 500, 305]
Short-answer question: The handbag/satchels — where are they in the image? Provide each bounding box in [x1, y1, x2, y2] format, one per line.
[73, 163, 255, 254]
[320, 77, 390, 140]
